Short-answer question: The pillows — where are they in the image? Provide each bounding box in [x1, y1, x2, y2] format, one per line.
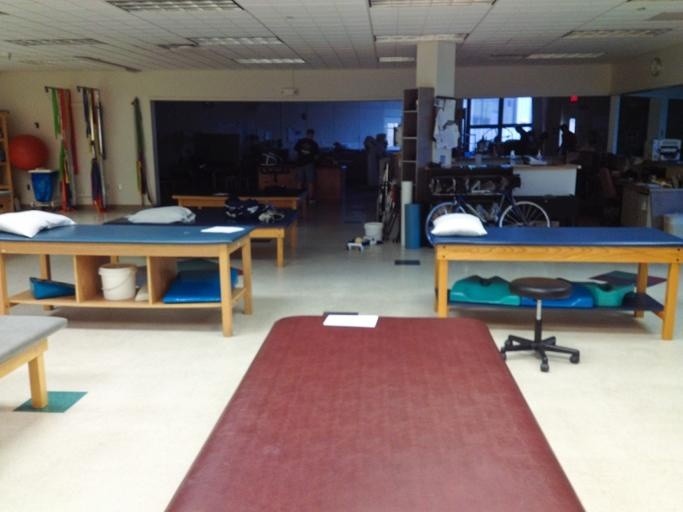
[431, 212, 487, 236]
[124, 206, 196, 226]
[0, 206, 77, 243]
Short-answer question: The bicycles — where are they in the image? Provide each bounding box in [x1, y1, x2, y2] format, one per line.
[418, 170, 553, 250]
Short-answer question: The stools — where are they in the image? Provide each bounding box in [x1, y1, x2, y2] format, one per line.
[498, 275, 582, 373]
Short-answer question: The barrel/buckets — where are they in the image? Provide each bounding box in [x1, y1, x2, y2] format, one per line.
[98, 262, 137, 301]
[663, 213, 683, 239]
[364, 222, 383, 240]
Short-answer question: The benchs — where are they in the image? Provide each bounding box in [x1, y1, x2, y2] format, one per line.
[1, 312, 69, 410]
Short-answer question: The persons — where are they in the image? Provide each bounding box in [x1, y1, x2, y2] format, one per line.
[488, 123, 573, 155]
[236, 129, 345, 211]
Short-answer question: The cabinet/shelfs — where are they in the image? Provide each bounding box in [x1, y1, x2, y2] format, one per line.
[620, 183, 682, 230]
[402, 86, 436, 202]
[0, 109, 13, 214]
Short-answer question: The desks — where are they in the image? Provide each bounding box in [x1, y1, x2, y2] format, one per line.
[499, 164, 587, 197]
[168, 313, 587, 510]
[101, 205, 299, 269]
[0, 222, 256, 336]
[172, 194, 307, 249]
[428, 224, 683, 343]
[257, 165, 341, 204]
